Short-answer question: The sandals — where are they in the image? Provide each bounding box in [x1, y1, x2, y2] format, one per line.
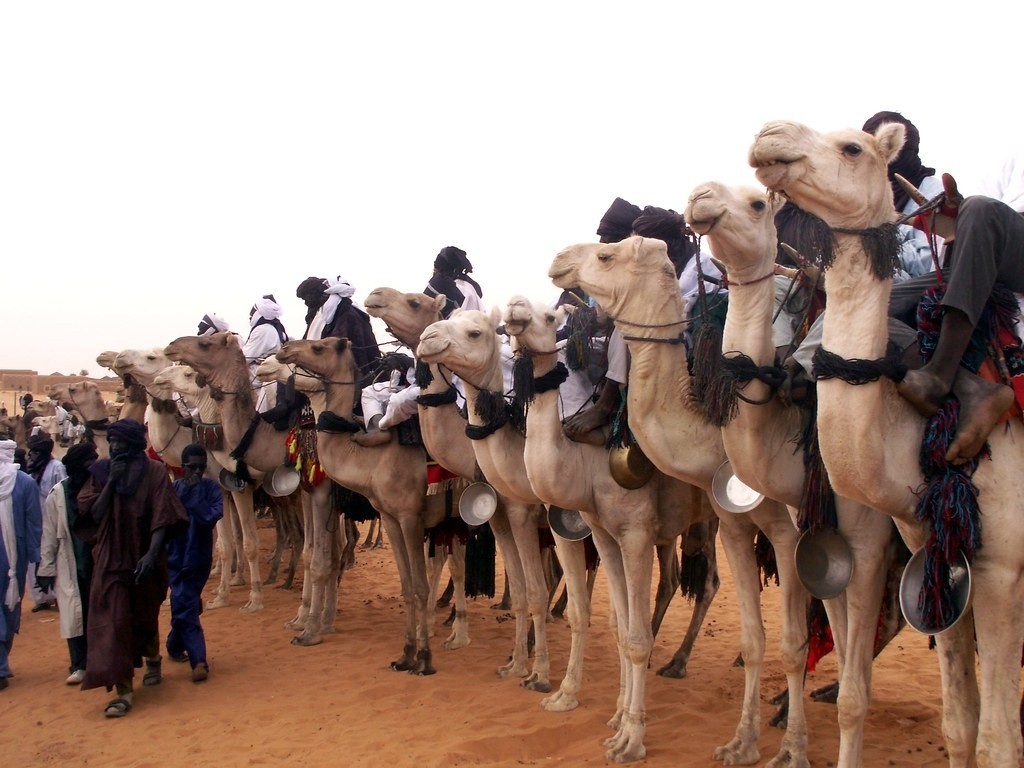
[143, 655, 162, 685]
[104, 699, 131, 718]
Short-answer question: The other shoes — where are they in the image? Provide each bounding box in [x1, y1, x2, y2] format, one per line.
[32, 603, 50, 612]
[0, 676, 8, 689]
[66, 669, 86, 683]
[191, 664, 208, 681]
[169, 647, 189, 663]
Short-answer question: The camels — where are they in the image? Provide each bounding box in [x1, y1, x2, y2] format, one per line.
[0, 120, 1024, 768]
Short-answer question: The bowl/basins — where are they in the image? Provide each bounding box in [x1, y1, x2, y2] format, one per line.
[459, 482, 498, 525]
[711, 460, 765, 514]
[263, 473, 280, 497]
[795, 526, 853, 600]
[272, 463, 300, 496]
[900, 543, 974, 635]
[547, 504, 592, 542]
[218, 468, 248, 492]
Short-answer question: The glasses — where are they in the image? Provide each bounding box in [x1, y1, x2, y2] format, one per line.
[184, 462, 208, 471]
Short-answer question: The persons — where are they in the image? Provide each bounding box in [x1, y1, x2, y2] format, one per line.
[0, 408, 67, 689]
[243, 294, 288, 372]
[36, 443, 100, 684]
[196, 314, 244, 348]
[165, 445, 224, 680]
[351, 248, 482, 448]
[451, 111, 1024, 465]
[78, 419, 187, 717]
[296, 276, 382, 367]
